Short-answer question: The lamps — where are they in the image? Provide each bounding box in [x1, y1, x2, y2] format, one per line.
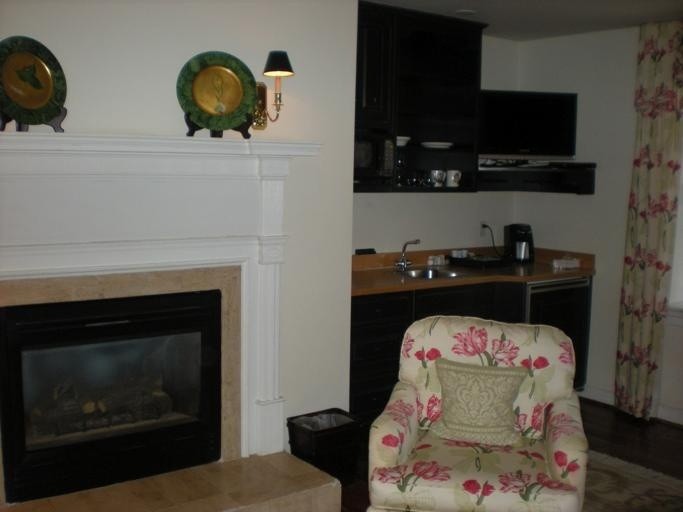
[252, 51, 295, 130]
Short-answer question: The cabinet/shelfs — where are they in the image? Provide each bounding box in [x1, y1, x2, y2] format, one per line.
[495, 277, 591, 391]
[353, 0, 597, 195]
[348, 282, 495, 419]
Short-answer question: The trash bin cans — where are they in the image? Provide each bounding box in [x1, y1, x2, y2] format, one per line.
[286, 407, 370, 512]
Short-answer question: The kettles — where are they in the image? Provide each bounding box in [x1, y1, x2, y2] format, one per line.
[503, 224, 535, 265]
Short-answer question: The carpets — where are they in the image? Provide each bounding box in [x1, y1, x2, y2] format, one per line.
[585, 449, 683, 512]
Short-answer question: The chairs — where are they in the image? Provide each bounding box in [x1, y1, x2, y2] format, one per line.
[367, 317, 589, 511]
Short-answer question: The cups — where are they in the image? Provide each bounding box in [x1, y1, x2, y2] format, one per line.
[431, 170, 462, 187]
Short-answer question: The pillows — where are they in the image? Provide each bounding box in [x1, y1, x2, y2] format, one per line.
[428, 360, 527, 446]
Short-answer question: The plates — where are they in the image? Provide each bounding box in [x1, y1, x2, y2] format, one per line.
[396, 136, 411, 146]
[0, 36, 67, 125]
[177, 50, 257, 131]
[422, 142, 453, 149]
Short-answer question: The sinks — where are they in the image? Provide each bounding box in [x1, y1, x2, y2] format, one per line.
[393, 264, 466, 281]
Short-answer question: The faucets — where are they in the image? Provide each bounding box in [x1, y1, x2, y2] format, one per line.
[396, 238, 421, 272]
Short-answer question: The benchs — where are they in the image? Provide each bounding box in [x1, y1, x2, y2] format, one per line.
[0, 452, 342, 512]
